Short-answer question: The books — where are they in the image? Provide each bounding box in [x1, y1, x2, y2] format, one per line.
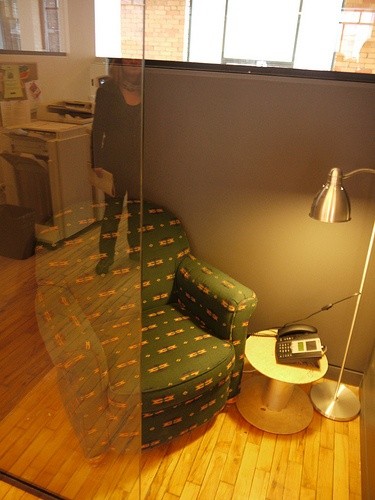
[94, 168, 115, 197]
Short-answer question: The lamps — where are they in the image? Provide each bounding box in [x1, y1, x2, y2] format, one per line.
[307, 167, 375, 422]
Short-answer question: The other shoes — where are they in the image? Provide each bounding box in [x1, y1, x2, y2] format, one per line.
[95, 257, 115, 275]
[129, 250, 142, 262]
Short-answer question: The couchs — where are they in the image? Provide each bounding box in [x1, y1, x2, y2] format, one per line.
[33, 196, 258, 467]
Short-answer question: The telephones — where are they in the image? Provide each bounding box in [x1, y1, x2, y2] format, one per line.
[275, 323, 324, 364]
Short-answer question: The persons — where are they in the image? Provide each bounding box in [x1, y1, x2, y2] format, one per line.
[89, 57, 142, 274]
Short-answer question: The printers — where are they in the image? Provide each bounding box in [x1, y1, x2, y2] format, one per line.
[0, 99, 111, 247]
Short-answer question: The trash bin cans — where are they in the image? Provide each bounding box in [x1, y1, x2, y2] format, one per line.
[0, 204, 37, 259]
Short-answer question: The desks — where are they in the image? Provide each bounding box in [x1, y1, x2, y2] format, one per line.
[234, 328, 328, 435]
